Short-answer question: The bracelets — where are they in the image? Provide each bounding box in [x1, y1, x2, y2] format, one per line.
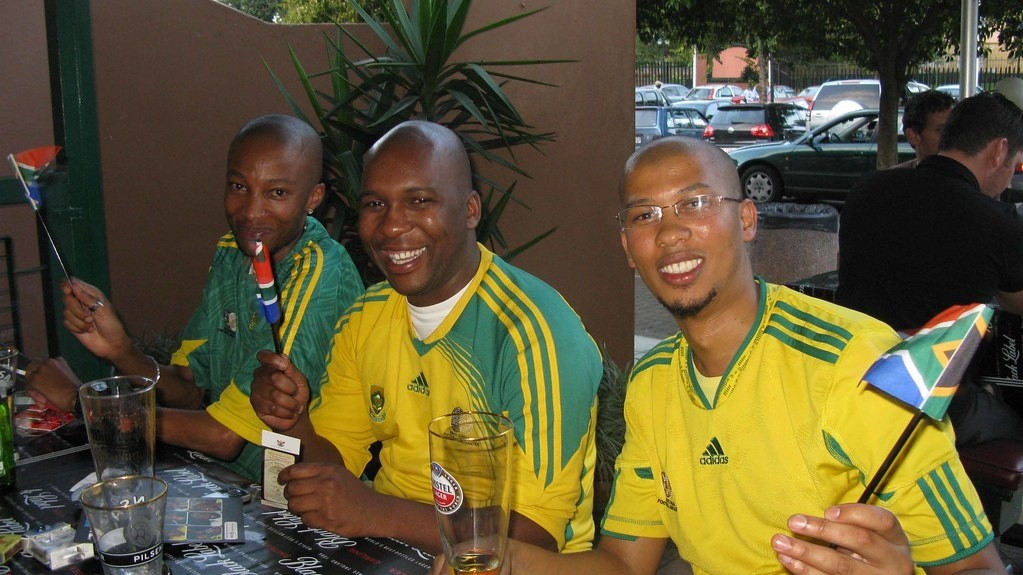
[110, 355, 159, 392]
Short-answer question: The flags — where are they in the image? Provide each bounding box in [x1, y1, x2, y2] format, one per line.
[857, 302, 998, 422]
[0, 146, 62, 213]
[246, 241, 283, 323]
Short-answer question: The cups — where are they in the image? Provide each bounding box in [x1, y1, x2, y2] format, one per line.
[428, 411, 514, 573]
[80, 476, 167, 575]
[78, 375, 154, 482]
[0, 346, 17, 496]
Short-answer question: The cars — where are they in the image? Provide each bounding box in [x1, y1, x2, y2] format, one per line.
[635, 82, 820, 149]
[725, 108, 1023, 206]
[935, 84, 985, 101]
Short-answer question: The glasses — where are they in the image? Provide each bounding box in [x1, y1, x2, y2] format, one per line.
[615, 193, 743, 230]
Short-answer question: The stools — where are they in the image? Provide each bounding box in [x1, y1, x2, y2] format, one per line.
[957, 437, 1023, 558]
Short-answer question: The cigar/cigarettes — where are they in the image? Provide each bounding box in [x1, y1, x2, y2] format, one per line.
[1, 363, 27, 377]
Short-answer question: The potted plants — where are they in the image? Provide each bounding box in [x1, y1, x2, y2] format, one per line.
[591, 337, 636, 536]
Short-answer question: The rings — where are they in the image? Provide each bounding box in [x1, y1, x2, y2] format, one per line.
[89, 298, 106, 311]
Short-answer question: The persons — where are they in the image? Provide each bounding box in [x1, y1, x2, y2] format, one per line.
[833, 91, 1022, 450]
[249, 119, 601, 559]
[436, 131, 1023, 574]
[21, 113, 368, 490]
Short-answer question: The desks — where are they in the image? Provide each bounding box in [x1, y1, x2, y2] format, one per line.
[785, 267, 1022, 385]
[0, 369, 458, 574]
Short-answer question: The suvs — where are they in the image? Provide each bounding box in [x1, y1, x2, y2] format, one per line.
[805, 77, 932, 142]
